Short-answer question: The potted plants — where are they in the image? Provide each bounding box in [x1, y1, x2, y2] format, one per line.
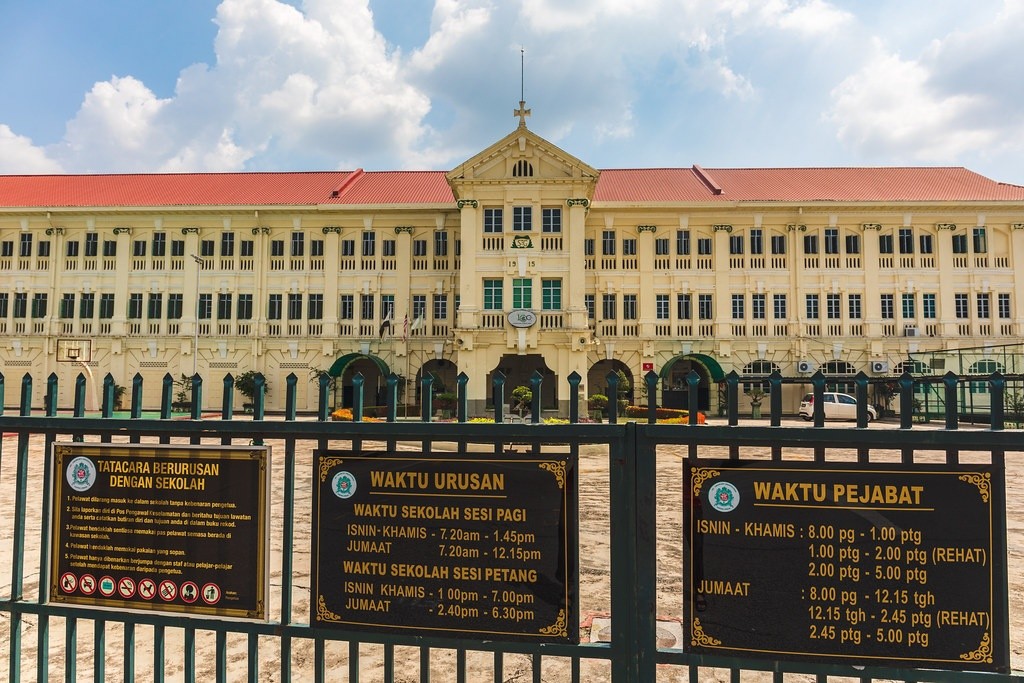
[746, 389, 769, 419]
[1003, 389, 1024, 429]
[881, 383, 897, 417]
[437, 393, 457, 419]
[589, 395, 608, 423]
[171, 373, 192, 412]
[912, 398, 925, 423]
[615, 369, 630, 417]
[113, 383, 126, 411]
[235, 370, 268, 414]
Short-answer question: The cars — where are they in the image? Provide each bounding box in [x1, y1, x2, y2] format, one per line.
[800, 392, 878, 423]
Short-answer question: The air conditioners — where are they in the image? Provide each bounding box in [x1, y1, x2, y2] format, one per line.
[871, 361, 888, 373]
[904, 328, 919, 337]
[797, 362, 812, 373]
